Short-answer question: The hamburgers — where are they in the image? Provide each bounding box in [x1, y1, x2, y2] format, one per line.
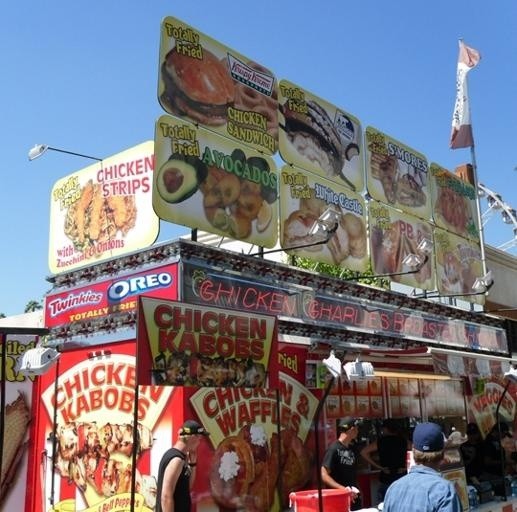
[161, 43, 238, 126]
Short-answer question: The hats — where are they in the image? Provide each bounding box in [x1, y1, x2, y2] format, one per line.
[180, 421, 209, 436]
[338, 416, 356, 432]
[495, 422, 512, 438]
[413, 423, 444, 451]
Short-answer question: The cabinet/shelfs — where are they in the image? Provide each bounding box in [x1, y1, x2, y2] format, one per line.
[439, 466, 470, 512]
[353, 470, 383, 508]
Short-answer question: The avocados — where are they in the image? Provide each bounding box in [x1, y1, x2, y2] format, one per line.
[157, 149, 277, 204]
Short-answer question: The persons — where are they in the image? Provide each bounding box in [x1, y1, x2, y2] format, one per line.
[359, 419, 412, 504]
[449, 421, 517, 486]
[155, 421, 210, 512]
[320, 415, 364, 511]
[383, 422, 462, 512]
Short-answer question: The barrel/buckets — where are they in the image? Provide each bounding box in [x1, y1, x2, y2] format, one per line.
[289, 489, 352, 511]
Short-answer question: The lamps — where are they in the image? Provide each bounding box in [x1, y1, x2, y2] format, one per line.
[413, 269, 495, 300]
[246, 206, 342, 257]
[342, 237, 435, 282]
[27, 143, 103, 163]
[323, 348, 375, 382]
[16, 347, 62, 375]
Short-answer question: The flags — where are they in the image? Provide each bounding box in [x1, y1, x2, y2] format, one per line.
[449, 41, 480, 150]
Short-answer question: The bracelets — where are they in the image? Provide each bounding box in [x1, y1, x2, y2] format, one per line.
[189, 463, 197, 467]
